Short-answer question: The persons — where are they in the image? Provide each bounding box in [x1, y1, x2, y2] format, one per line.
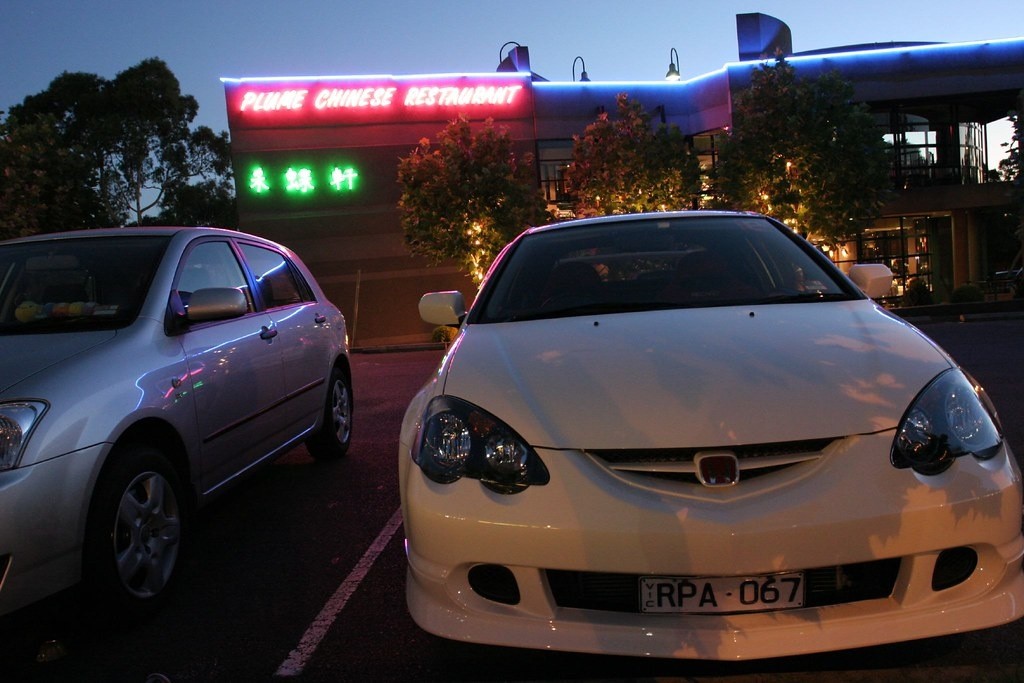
[892, 270, 912, 286]
[582, 250, 609, 282]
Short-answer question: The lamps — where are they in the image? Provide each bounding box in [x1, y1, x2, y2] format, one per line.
[572, 56, 590, 83]
[665, 48, 681, 81]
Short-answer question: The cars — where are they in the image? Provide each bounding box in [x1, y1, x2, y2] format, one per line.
[399, 211, 1023, 681]
[0, 228, 357, 623]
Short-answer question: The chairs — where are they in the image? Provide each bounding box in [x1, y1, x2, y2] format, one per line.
[673, 248, 748, 303]
